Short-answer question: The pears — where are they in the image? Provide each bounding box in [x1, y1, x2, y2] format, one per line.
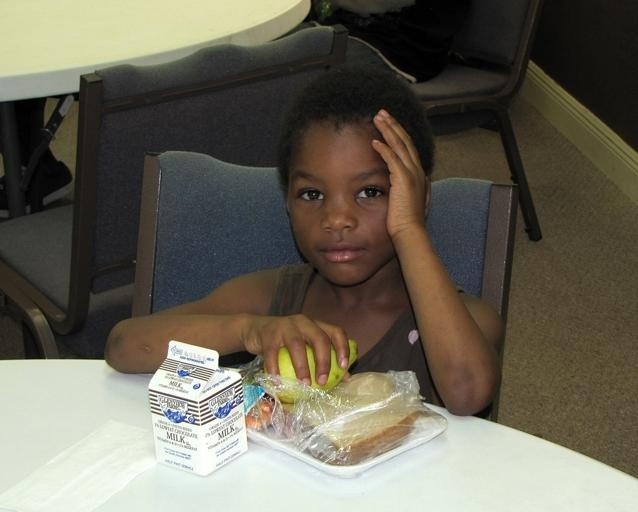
[257, 338, 358, 404]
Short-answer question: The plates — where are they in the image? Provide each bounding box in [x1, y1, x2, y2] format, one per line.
[247, 407, 449, 479]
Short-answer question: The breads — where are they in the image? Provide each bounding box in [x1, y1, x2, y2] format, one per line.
[279, 372, 422, 464]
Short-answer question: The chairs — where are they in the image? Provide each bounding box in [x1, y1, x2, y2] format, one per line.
[1, 24, 348, 360]
[130, 149, 520, 423]
[410, 0, 547, 242]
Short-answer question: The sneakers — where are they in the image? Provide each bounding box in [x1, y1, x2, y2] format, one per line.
[0, 160, 72, 219]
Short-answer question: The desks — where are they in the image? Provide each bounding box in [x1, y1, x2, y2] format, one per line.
[1, 1, 313, 219]
[0, 359, 638, 512]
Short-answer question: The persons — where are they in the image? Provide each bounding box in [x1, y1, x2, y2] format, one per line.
[102, 65, 507, 422]
